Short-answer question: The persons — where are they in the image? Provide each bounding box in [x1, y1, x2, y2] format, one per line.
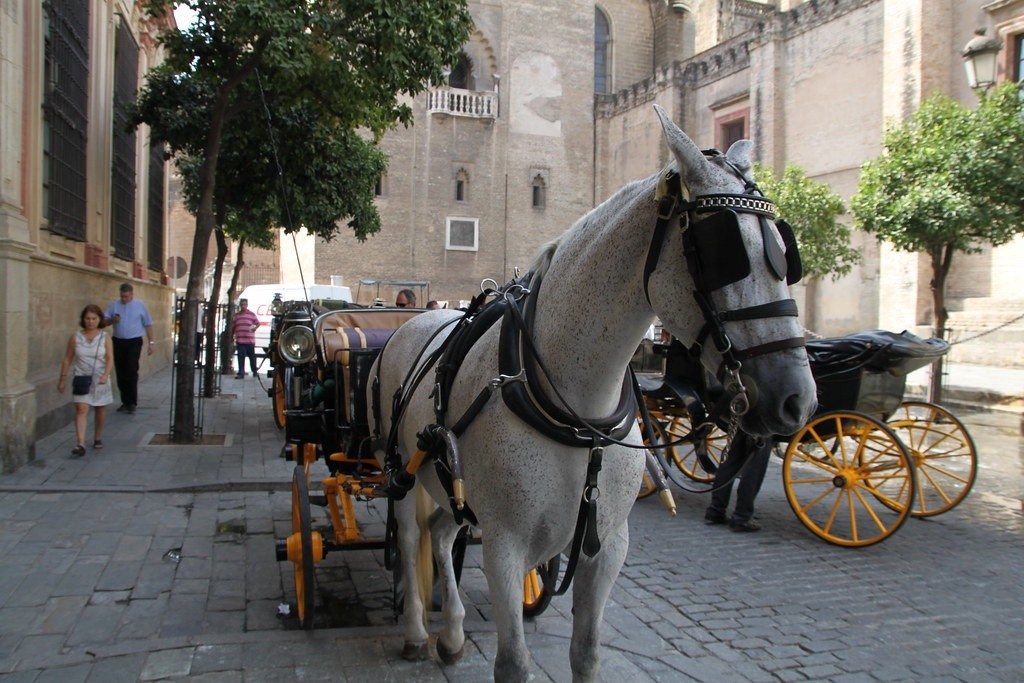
[396, 289, 416, 308]
[56, 305, 114, 454]
[705, 419, 774, 533]
[100, 283, 155, 412]
[425, 301, 441, 308]
[231, 298, 261, 379]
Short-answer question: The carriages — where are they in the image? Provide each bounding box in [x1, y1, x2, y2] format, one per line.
[264, 105, 820, 683]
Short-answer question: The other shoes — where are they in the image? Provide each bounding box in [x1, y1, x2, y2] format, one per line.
[116, 403, 137, 414]
[235, 374, 244, 379]
[730, 518, 762, 532]
[253, 372, 258, 377]
[71, 445, 85, 456]
[704, 507, 733, 522]
[94, 440, 104, 448]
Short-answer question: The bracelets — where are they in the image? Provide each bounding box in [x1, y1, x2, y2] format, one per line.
[61, 374, 67, 376]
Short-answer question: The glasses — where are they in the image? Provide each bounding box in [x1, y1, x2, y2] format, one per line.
[396, 302, 412, 308]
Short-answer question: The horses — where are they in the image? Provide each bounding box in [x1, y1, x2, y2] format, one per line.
[367, 103, 818, 683]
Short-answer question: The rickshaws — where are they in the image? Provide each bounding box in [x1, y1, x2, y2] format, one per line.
[625, 324, 981, 547]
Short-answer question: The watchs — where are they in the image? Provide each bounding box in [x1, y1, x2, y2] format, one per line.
[148, 340, 154, 344]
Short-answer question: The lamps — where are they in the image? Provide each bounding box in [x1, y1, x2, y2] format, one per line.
[961, 27, 1002, 100]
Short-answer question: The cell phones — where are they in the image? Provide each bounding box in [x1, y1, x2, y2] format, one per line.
[116, 314, 120, 323]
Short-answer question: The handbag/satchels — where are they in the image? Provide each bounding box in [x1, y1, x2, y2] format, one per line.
[72, 376, 93, 395]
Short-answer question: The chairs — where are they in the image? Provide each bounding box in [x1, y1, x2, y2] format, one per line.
[316, 309, 430, 413]
